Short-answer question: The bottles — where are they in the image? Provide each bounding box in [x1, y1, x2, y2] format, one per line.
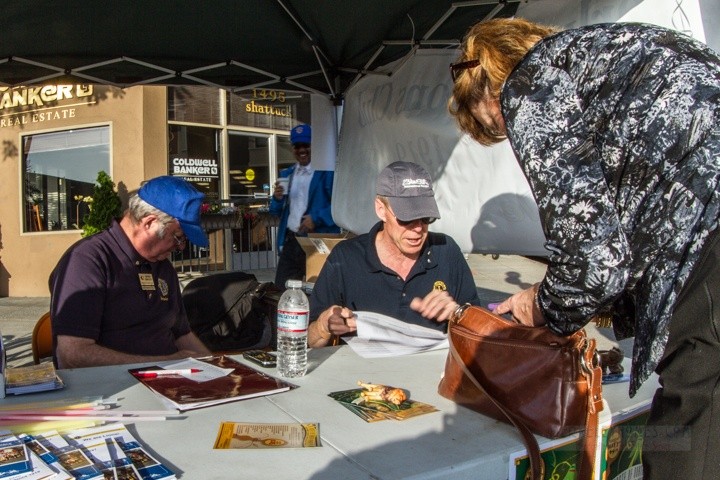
[277, 280, 310, 377]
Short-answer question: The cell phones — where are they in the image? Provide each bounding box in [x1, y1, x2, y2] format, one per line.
[243, 350, 276, 368]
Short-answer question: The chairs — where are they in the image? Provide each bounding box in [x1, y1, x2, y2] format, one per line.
[31, 312, 54, 365]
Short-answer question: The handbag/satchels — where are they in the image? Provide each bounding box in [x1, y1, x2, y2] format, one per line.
[438, 304, 598, 439]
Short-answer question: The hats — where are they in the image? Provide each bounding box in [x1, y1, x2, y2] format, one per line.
[139, 176, 209, 246]
[377, 161, 441, 221]
[291, 125, 311, 144]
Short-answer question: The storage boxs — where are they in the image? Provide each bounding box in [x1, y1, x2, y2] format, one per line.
[294, 232, 353, 283]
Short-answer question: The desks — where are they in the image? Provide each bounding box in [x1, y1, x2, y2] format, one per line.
[0, 323, 662, 480]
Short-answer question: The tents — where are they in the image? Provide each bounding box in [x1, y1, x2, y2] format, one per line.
[0, 0, 720, 263]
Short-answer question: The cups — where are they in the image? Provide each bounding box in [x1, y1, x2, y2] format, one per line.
[277, 177, 290, 195]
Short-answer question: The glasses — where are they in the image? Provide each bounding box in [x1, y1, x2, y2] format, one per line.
[380, 200, 436, 226]
[293, 141, 311, 150]
[450, 58, 482, 82]
[163, 224, 187, 245]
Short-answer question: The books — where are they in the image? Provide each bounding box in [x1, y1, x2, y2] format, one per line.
[0, 423, 177, 480]
[4, 361, 64, 395]
[128, 354, 290, 410]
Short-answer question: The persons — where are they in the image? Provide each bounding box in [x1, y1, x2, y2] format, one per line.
[48, 175, 213, 369]
[270, 125, 341, 290]
[448, 16, 720, 480]
[307, 160, 482, 349]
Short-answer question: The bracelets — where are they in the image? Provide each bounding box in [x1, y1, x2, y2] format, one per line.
[316, 314, 332, 341]
